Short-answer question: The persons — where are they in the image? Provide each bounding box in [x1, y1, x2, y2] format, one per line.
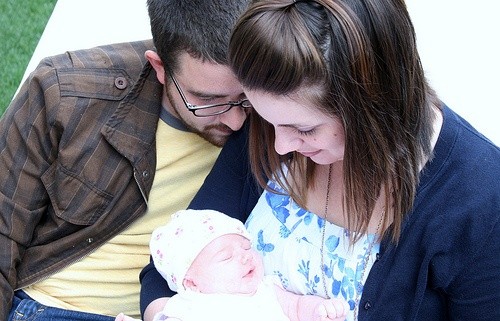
[115, 209, 350, 321]
[133, 0, 500, 321]
[0, 0, 254, 321]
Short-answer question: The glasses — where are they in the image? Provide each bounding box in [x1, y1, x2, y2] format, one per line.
[158, 53, 253, 117]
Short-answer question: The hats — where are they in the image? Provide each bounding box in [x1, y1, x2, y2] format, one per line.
[149, 209, 251, 293]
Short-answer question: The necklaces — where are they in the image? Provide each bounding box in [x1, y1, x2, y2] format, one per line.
[321, 164, 390, 321]
